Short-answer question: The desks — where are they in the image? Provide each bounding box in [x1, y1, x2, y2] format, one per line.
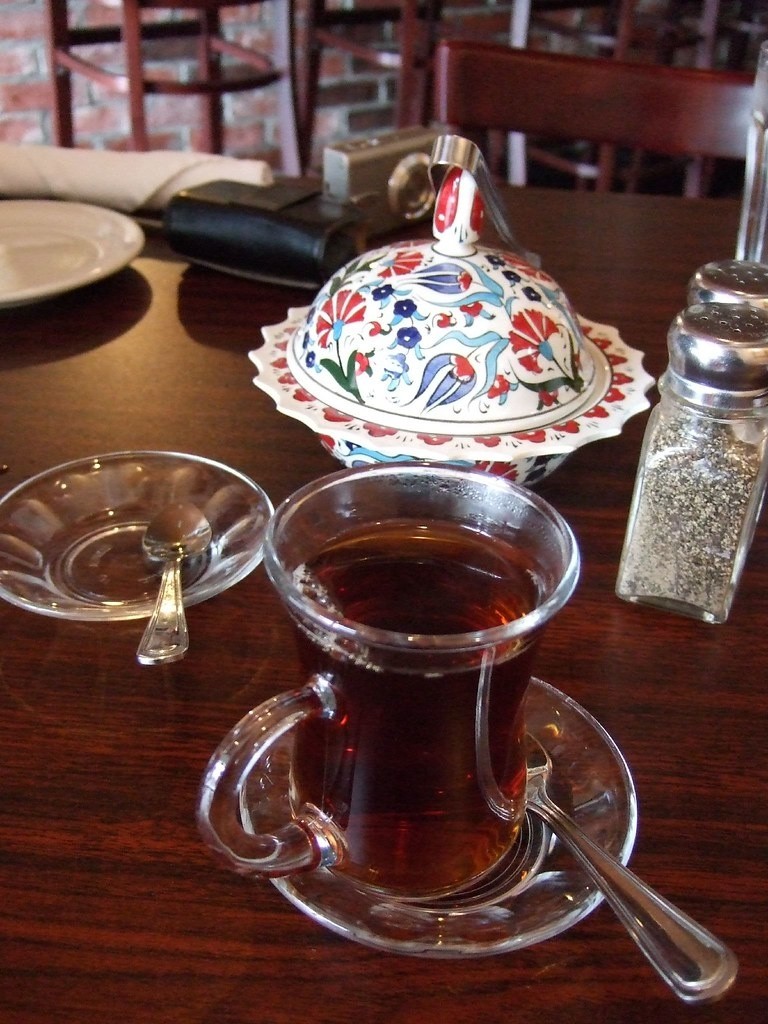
[0, 184, 768, 1024]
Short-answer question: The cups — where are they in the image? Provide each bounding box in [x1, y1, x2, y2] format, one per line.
[197, 462, 580, 894]
[735, 40, 768, 264]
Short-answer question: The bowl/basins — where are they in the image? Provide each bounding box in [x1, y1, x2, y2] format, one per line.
[249, 169, 656, 487]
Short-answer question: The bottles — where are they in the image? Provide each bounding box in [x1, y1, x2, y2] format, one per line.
[686, 260, 768, 548]
[615, 303, 768, 623]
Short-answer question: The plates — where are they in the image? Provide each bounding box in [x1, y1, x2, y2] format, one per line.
[240, 676, 638, 956]
[0, 451, 274, 622]
[0, 199, 146, 307]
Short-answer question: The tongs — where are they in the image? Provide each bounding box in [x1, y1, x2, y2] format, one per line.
[427, 135, 542, 267]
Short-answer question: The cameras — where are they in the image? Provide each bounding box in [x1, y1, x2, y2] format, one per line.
[325, 125, 458, 233]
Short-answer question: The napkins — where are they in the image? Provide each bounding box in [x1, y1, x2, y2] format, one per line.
[0, 143, 275, 214]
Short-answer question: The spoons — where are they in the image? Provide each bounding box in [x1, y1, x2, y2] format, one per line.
[136, 500, 212, 666]
[523, 729, 740, 1007]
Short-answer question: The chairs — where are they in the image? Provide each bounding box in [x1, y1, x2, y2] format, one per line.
[431, 37, 762, 204]
